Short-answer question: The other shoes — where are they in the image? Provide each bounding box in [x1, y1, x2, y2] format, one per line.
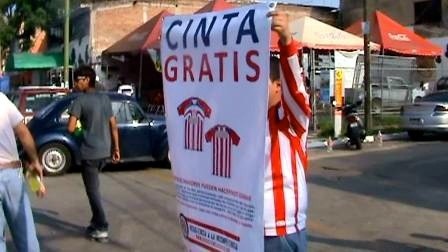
[86, 226, 108, 242]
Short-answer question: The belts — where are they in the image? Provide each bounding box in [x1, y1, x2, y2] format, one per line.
[0, 160, 21, 169]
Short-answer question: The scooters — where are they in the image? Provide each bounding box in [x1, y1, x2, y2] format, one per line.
[335, 100, 367, 151]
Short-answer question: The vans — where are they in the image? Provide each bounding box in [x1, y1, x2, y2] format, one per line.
[12, 85, 71, 117]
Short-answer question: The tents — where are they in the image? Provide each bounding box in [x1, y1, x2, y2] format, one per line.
[102, 0, 443, 136]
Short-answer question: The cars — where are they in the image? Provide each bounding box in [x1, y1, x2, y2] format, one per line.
[399, 91, 448, 140]
[26, 95, 174, 176]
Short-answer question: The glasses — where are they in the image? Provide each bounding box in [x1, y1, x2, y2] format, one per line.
[75, 78, 87, 83]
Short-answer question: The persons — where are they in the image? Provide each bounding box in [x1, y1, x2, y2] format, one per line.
[0, 93, 44, 252]
[67, 67, 119, 243]
[262, 10, 312, 252]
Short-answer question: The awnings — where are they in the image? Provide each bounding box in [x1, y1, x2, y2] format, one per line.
[5, 52, 71, 71]
